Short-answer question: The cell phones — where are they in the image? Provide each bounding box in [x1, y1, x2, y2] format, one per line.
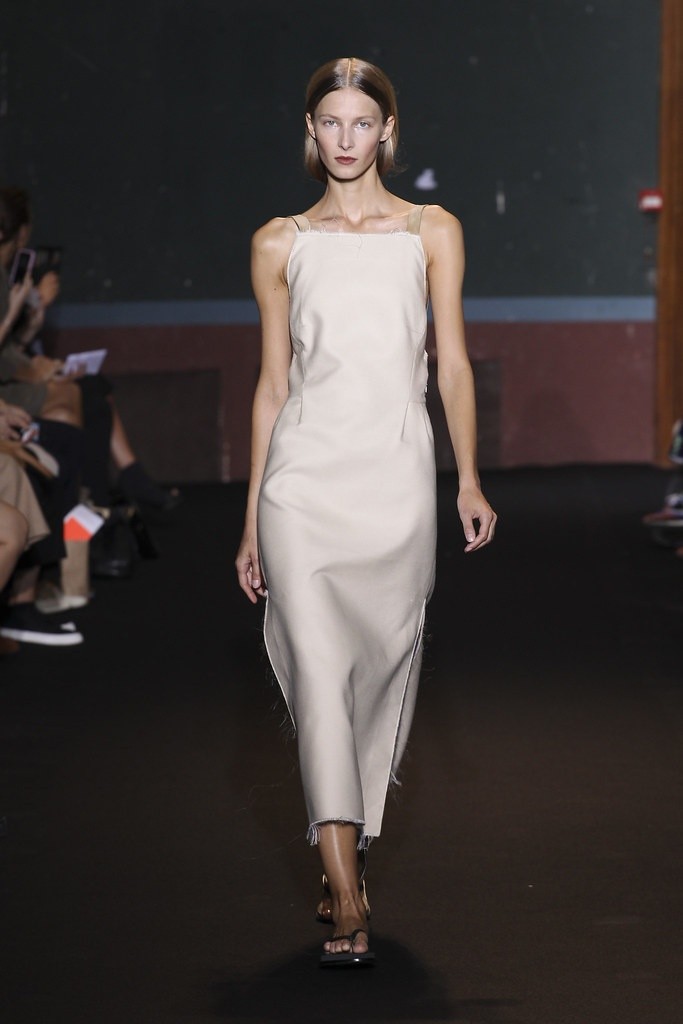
[8, 250, 35, 287]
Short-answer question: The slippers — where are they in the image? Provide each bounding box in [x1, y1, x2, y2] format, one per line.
[319, 928, 375, 961]
[318, 873, 368, 923]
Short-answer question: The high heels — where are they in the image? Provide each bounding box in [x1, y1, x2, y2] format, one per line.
[116, 463, 185, 518]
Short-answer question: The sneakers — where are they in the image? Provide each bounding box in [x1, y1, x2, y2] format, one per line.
[1, 600, 85, 645]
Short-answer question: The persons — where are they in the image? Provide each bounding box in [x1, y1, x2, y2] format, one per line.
[235, 56, 498, 963]
[0, 190, 183, 648]
[645, 421, 682, 531]
